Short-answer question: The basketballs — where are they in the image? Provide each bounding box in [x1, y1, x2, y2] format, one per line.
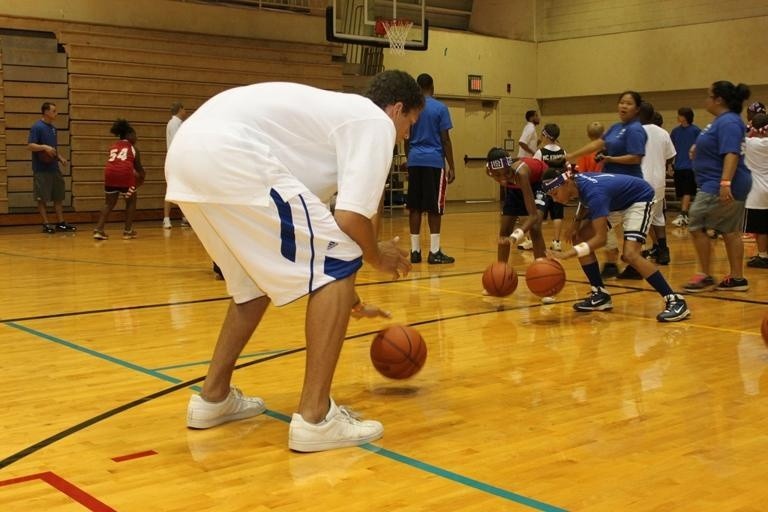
[762, 311, 768, 345]
[483, 262, 518, 295]
[526, 256, 566, 297]
[370, 326, 427, 379]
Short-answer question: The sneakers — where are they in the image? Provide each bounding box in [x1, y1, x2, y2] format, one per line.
[713, 277, 749, 291]
[410, 249, 421, 263]
[600, 245, 670, 280]
[288, 396, 384, 453]
[671, 214, 686, 227]
[656, 294, 690, 322]
[187, 386, 268, 428]
[43, 218, 189, 240]
[517, 240, 533, 250]
[427, 250, 455, 264]
[747, 254, 768, 268]
[550, 240, 562, 251]
[681, 273, 716, 292]
[573, 286, 613, 312]
[684, 215, 690, 224]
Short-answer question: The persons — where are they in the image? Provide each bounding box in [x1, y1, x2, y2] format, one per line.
[164, 69, 426, 454]
[542, 157, 690, 320]
[516, 111, 567, 252]
[403, 73, 456, 265]
[567, 80, 768, 292]
[162, 101, 191, 229]
[27, 101, 77, 234]
[92, 119, 146, 241]
[486, 147, 556, 303]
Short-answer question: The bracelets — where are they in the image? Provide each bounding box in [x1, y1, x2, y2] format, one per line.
[510, 228, 524, 241]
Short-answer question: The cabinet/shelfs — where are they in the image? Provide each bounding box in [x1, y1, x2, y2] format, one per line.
[382, 140, 409, 217]
